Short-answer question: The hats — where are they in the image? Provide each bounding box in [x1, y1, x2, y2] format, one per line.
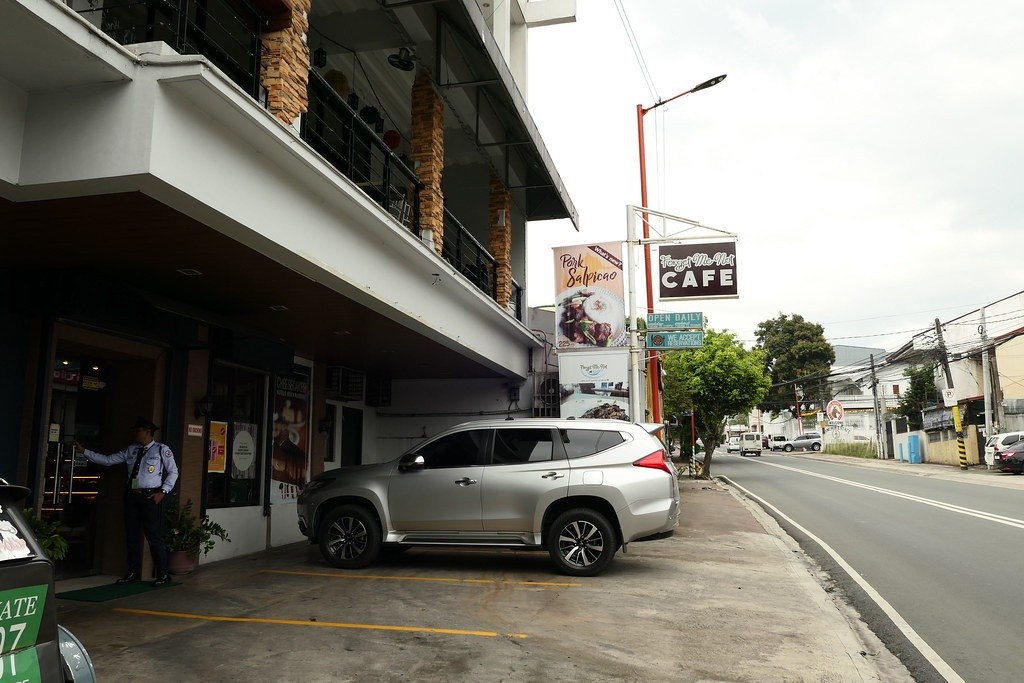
[130, 416, 159, 433]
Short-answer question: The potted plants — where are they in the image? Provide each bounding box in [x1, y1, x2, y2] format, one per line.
[163, 494, 231, 575]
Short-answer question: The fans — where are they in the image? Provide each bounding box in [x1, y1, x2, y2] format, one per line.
[388, 47, 418, 71]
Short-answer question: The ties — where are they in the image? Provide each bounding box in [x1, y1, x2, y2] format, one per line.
[126, 448, 144, 491]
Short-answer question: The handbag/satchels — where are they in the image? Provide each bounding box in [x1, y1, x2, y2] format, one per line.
[141, 487, 173, 513]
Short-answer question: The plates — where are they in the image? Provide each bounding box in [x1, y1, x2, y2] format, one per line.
[555, 286, 627, 347]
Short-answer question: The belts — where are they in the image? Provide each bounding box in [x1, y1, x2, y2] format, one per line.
[126, 488, 142, 493]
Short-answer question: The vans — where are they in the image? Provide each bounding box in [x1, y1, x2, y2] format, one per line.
[739, 432, 762, 456]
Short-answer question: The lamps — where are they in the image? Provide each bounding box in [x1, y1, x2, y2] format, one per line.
[347, 54, 359, 110]
[314, 33, 327, 68]
[375, 105, 384, 134]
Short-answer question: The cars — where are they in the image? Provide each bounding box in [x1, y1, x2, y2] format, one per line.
[762, 435, 788, 451]
[984, 431, 1024, 473]
[726, 437, 739, 453]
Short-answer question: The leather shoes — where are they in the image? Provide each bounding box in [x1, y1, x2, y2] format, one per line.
[116, 569, 141, 584]
[154, 569, 168, 584]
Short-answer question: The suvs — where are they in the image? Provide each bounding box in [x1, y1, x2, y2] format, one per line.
[785, 432, 822, 451]
[297, 417, 681, 575]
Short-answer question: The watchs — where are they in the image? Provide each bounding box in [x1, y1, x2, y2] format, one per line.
[160, 489, 168, 495]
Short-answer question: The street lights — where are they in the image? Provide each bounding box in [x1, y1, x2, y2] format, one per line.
[634, 74, 730, 441]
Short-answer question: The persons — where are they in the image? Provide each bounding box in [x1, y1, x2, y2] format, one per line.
[72, 420, 179, 585]
